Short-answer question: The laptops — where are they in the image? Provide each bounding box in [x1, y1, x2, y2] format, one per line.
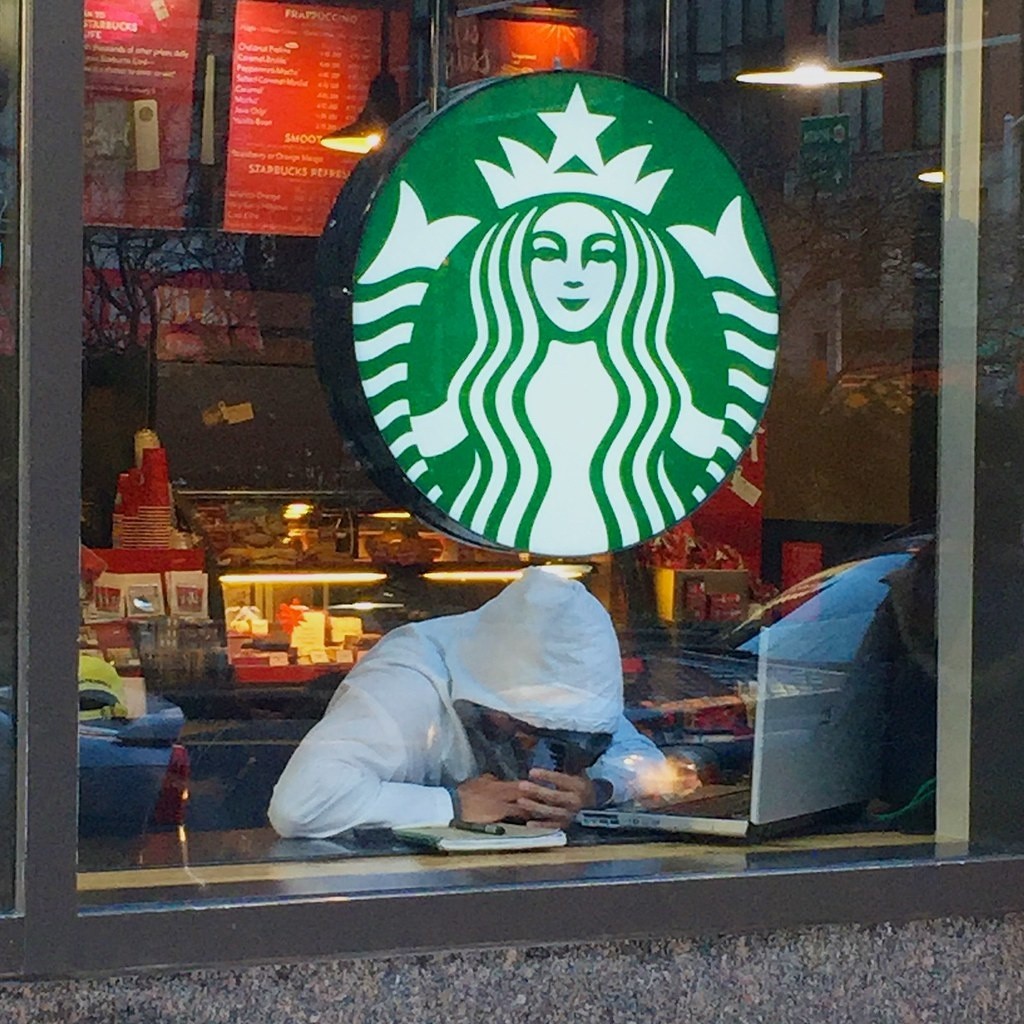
[576, 608, 898, 842]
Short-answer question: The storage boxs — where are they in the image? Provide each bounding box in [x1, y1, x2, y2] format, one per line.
[645, 562, 750, 624]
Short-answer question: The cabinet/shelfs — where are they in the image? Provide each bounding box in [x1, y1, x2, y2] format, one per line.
[175, 487, 598, 696]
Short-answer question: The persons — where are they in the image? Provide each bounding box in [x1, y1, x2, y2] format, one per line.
[265, 567, 675, 839]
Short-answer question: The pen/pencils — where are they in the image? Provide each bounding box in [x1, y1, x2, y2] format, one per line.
[451, 819, 505, 835]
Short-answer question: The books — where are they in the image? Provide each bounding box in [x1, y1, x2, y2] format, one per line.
[395, 820, 568, 850]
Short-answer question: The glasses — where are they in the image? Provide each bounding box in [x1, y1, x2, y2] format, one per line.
[506, 716, 538, 735]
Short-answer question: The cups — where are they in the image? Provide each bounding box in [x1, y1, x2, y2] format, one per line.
[112, 448, 171, 550]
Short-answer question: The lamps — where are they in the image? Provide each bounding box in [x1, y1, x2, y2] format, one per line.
[319, 0, 401, 153]
[734, 0, 884, 89]
